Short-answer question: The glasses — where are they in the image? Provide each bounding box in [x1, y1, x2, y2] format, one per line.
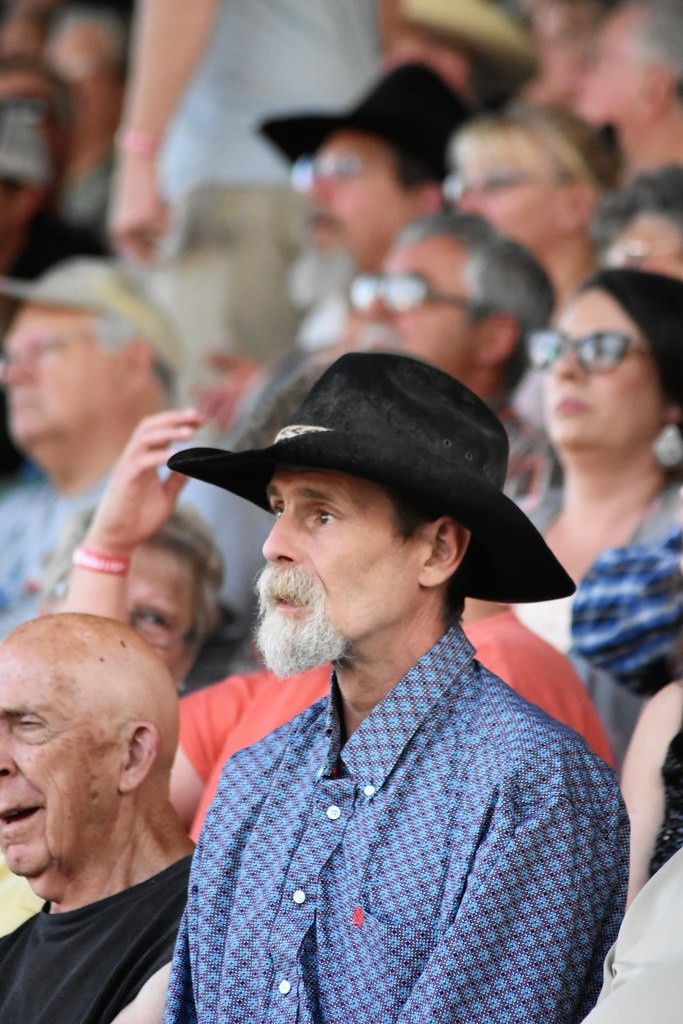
[287, 156, 392, 194]
[441, 166, 566, 202]
[526, 327, 656, 374]
[350, 271, 494, 318]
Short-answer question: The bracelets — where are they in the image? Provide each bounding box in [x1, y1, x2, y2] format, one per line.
[73, 547, 130, 577]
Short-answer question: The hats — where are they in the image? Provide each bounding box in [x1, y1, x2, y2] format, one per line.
[403, 0, 537, 78]
[579, 270, 683, 406]
[260, 62, 465, 184]
[0, 256, 176, 345]
[166, 351, 576, 604]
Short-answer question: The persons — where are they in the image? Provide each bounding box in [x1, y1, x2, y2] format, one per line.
[578, 846, 683, 1023]
[0, 1, 683, 895]
[161, 351, 632, 1024]
[66, 365, 611, 847]
[0, 613, 199, 1024]
